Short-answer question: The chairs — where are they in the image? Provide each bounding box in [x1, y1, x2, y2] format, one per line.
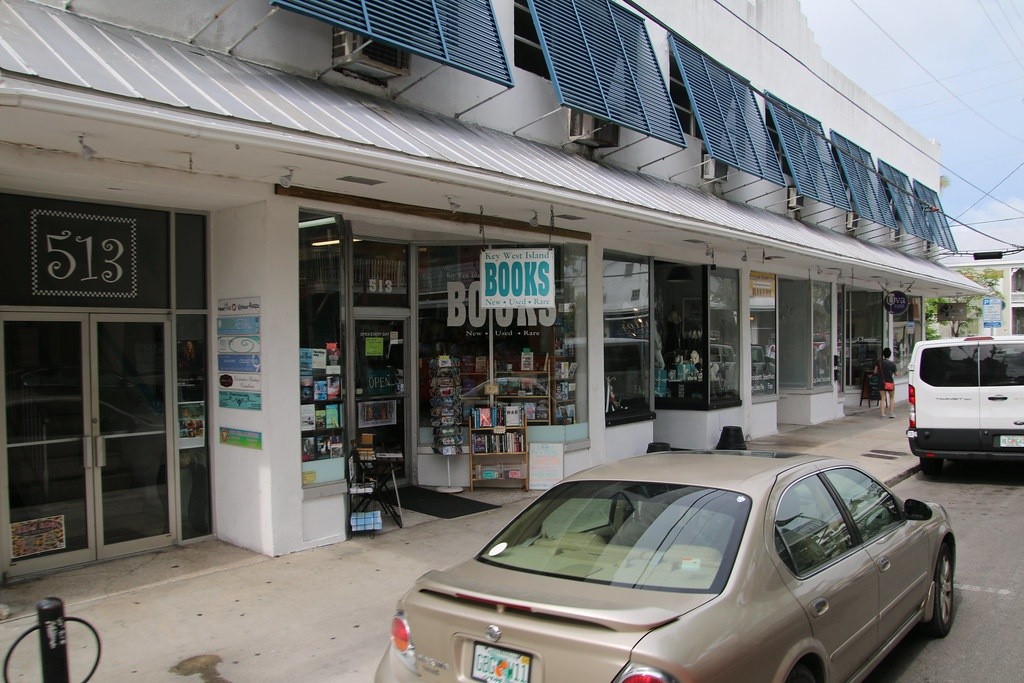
[667, 544, 722, 561]
[348, 439, 404, 528]
[556, 531, 605, 545]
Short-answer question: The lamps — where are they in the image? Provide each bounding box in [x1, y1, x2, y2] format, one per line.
[529, 212, 539, 227]
[741, 250, 748, 261]
[447, 197, 462, 214]
[706, 245, 713, 256]
[817, 265, 823, 275]
[281, 170, 295, 189]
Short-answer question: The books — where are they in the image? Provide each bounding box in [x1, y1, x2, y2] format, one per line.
[300, 348, 346, 458]
[472, 432, 525, 453]
[471, 405, 526, 428]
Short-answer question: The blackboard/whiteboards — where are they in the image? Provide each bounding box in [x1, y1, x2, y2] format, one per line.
[865, 372, 882, 400]
[529, 443, 563, 490]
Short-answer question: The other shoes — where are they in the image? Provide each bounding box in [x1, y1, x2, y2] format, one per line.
[889, 413, 896, 417]
[881, 415, 887, 417]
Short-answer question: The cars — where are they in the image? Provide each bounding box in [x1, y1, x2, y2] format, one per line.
[709, 344, 736, 384]
[374, 450, 957, 683]
[6, 397, 176, 496]
[460, 377, 563, 407]
[851, 344, 897, 374]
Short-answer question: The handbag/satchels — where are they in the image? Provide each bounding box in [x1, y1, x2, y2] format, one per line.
[885, 382, 894, 390]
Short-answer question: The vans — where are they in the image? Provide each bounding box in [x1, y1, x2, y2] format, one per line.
[906, 335, 1024, 475]
[750, 346, 766, 382]
[563, 337, 666, 399]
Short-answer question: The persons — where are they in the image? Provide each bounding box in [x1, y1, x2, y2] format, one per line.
[874, 348, 907, 419]
[183, 340, 203, 372]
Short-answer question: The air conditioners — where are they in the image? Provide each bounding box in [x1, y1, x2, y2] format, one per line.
[846, 211, 859, 230]
[889, 227, 901, 242]
[922, 240, 931, 252]
[703, 153, 728, 183]
[787, 187, 806, 210]
[332, 23, 413, 81]
[570, 108, 621, 149]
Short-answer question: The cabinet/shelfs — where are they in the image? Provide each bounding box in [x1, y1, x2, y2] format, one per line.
[492, 353, 552, 426]
[456, 356, 489, 426]
[467, 404, 530, 492]
[543, 320, 576, 425]
